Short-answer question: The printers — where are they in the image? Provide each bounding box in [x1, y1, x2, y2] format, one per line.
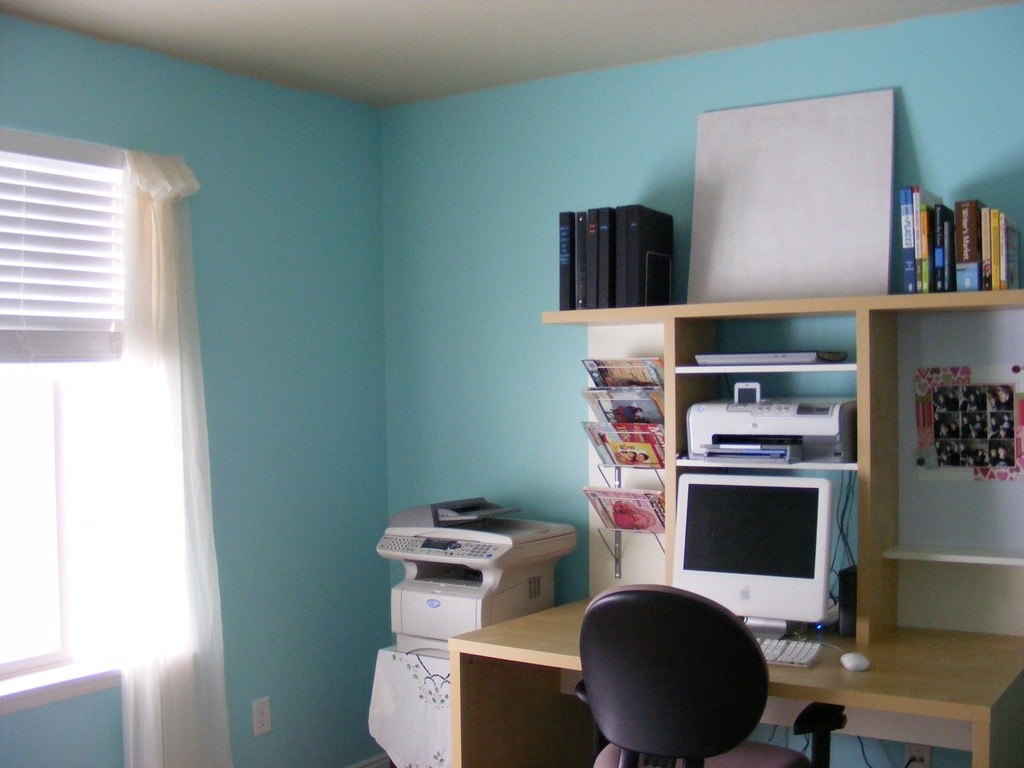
[375, 496, 574, 661]
[686, 382, 857, 463]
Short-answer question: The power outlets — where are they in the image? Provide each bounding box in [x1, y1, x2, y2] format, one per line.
[904, 743, 930, 768]
[252, 696, 271, 736]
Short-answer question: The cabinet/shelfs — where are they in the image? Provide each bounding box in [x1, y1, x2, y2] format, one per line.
[448, 289, 1024, 768]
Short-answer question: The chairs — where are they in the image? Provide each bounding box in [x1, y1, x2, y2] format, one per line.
[576, 584, 846, 768]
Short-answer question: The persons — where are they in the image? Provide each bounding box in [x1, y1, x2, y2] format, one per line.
[934, 384, 1014, 466]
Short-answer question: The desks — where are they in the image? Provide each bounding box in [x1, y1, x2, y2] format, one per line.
[368, 644, 451, 768]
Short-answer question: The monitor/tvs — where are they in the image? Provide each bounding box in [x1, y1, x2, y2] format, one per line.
[674, 473, 833, 641]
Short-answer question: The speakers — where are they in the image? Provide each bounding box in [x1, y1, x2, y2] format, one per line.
[838, 565, 858, 637]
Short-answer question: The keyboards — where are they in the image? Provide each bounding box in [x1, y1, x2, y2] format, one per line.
[754, 636, 821, 668]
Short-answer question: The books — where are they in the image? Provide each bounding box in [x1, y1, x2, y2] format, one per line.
[900, 185, 1020, 295]
[578, 357, 665, 532]
[558, 204, 674, 311]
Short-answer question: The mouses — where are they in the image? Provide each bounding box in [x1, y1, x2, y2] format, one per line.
[839, 653, 870, 671]
